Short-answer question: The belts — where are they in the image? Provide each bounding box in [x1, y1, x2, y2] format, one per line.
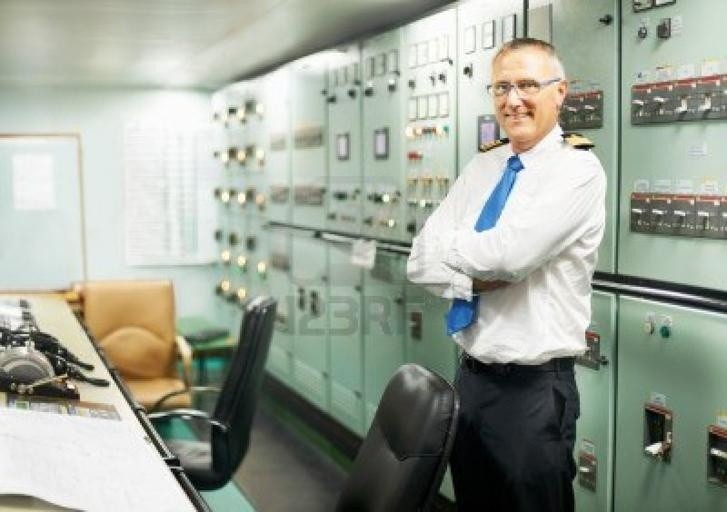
[459, 351, 575, 377]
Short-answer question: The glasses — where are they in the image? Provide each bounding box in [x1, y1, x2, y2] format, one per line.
[486, 77, 559, 98]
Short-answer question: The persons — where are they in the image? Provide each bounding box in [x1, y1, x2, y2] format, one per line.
[403, 37, 607, 512]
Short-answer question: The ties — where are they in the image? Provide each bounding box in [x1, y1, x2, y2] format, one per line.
[443, 154, 524, 336]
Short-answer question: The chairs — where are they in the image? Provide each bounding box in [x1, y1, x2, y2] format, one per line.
[143, 293, 281, 492]
[348, 360, 460, 511]
[86, 281, 198, 415]
